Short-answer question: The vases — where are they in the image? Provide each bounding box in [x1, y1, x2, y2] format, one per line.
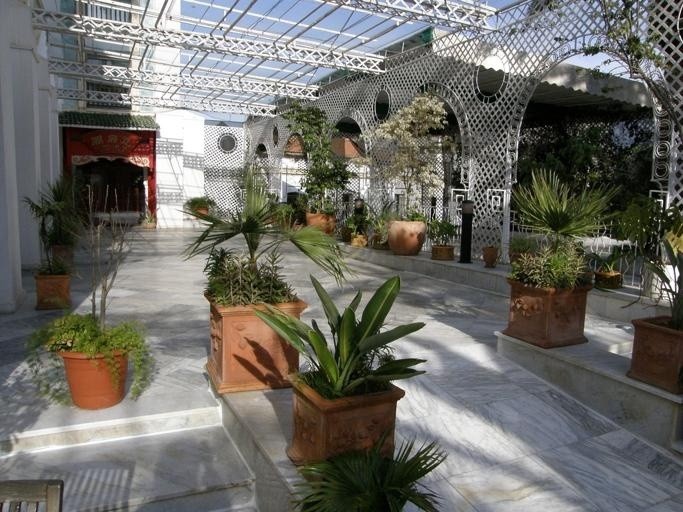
[482, 245, 498, 269]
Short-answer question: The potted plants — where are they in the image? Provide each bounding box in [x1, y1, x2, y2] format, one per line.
[248, 273, 433, 477]
[502, 167, 625, 349]
[427, 217, 457, 261]
[585, 244, 632, 289]
[177, 170, 347, 395]
[17, 183, 158, 410]
[606, 194, 683, 397]
[340, 196, 428, 255]
